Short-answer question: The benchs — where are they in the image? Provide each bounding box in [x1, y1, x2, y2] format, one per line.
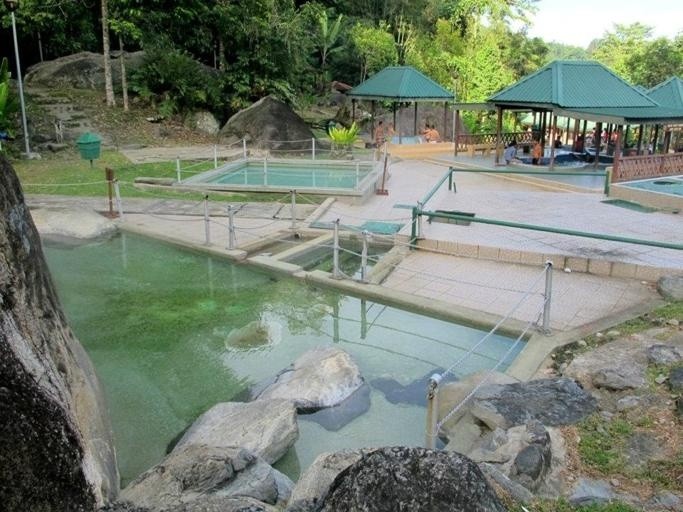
[467, 140, 550, 161]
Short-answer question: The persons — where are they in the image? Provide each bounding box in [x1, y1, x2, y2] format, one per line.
[418, 124, 440, 143]
[503, 125, 657, 169]
[386, 122, 395, 137]
[373, 121, 385, 146]
